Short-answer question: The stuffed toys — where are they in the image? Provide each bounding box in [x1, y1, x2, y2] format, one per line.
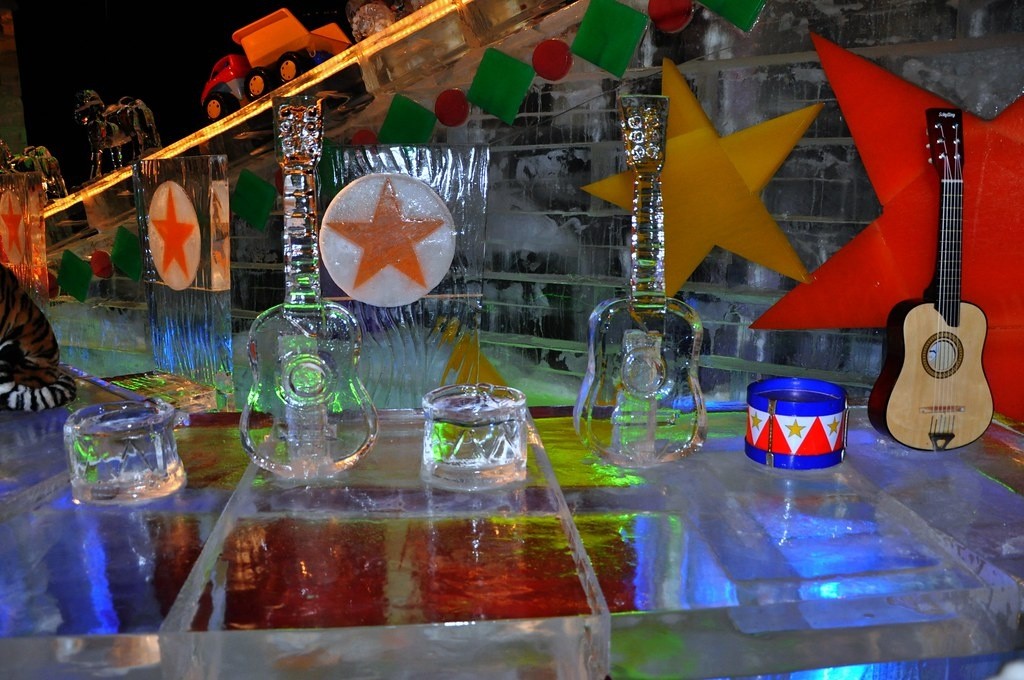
[0, 263, 76, 410]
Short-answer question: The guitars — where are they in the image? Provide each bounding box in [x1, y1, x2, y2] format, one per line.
[238, 95, 381, 479]
[572, 92, 709, 469]
[866, 105, 994, 451]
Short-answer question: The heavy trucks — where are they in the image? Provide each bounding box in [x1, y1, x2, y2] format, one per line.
[199, 8, 354, 123]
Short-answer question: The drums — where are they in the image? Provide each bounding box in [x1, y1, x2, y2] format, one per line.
[746, 376, 851, 470]
[63, 401, 187, 506]
[422, 384, 527, 473]
